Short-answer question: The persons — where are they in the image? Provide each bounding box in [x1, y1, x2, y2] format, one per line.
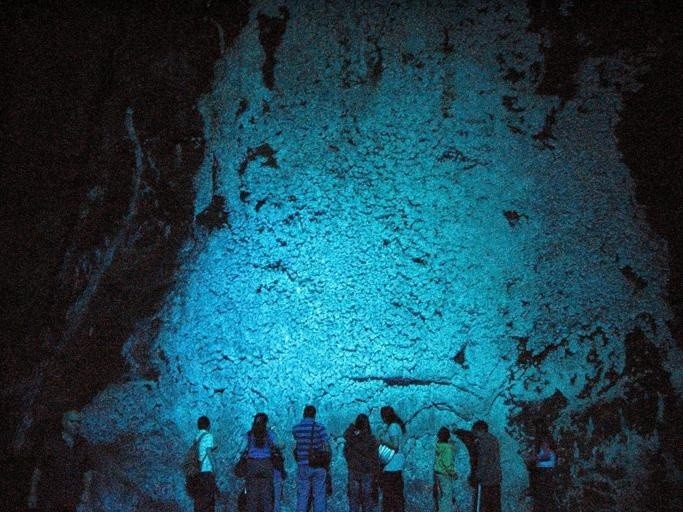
[376, 405, 408, 512]
[430, 427, 461, 511]
[343, 414, 380, 511]
[237, 412, 286, 510]
[191, 414, 221, 511]
[468, 421, 502, 512]
[519, 418, 558, 511]
[291, 405, 331, 511]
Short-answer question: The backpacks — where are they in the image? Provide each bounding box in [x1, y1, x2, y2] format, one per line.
[184, 432, 210, 479]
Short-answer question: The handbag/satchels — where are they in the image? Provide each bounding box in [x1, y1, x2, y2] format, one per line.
[475, 463, 502, 488]
[234, 451, 249, 478]
[270, 443, 286, 471]
[378, 444, 396, 465]
[309, 447, 333, 467]
